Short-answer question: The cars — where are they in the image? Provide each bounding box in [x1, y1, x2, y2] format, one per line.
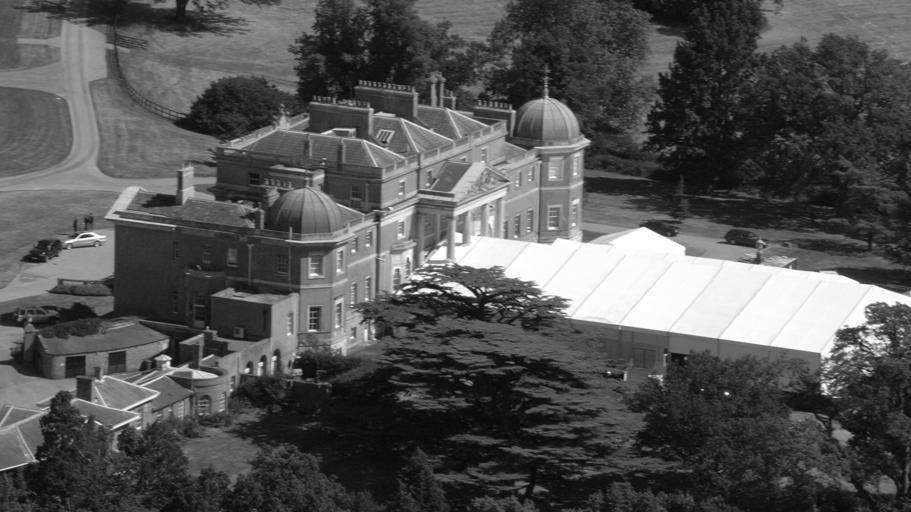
[28, 239, 62, 263]
[725, 229, 769, 249]
[640, 219, 680, 237]
[62, 231, 107, 250]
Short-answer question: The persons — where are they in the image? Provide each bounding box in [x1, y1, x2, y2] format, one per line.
[72, 211, 94, 234]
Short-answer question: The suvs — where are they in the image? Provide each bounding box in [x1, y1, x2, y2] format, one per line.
[13, 305, 61, 325]
